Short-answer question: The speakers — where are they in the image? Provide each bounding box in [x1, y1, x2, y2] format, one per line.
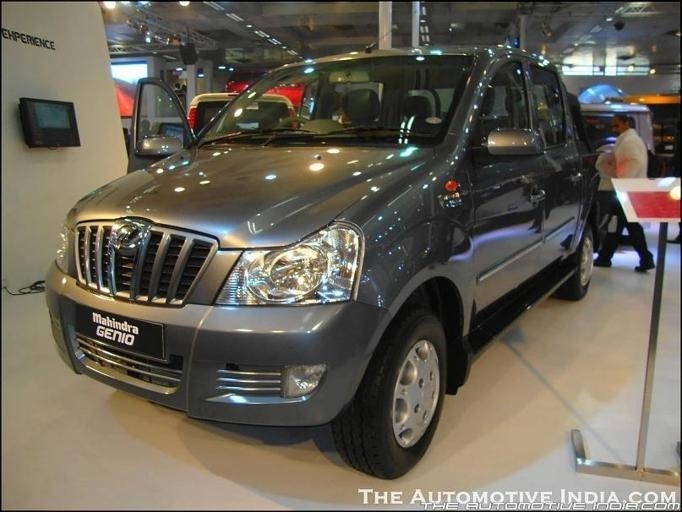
[179, 43, 197, 64]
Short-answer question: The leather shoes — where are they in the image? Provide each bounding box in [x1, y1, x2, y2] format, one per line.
[666, 238, 682, 243]
[593, 258, 612, 267]
[634, 263, 655, 272]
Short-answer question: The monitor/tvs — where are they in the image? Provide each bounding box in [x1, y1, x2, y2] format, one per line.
[19, 98, 80, 147]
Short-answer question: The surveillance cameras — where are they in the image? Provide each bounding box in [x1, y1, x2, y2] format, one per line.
[613, 18, 626, 30]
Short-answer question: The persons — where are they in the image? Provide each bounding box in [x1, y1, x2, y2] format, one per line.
[592, 109, 657, 272]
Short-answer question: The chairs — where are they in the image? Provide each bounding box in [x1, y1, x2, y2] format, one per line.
[342, 89, 431, 129]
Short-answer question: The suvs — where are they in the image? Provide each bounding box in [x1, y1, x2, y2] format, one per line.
[38, 39, 662, 481]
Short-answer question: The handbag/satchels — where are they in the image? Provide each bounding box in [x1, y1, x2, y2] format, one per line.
[647, 149, 666, 177]
